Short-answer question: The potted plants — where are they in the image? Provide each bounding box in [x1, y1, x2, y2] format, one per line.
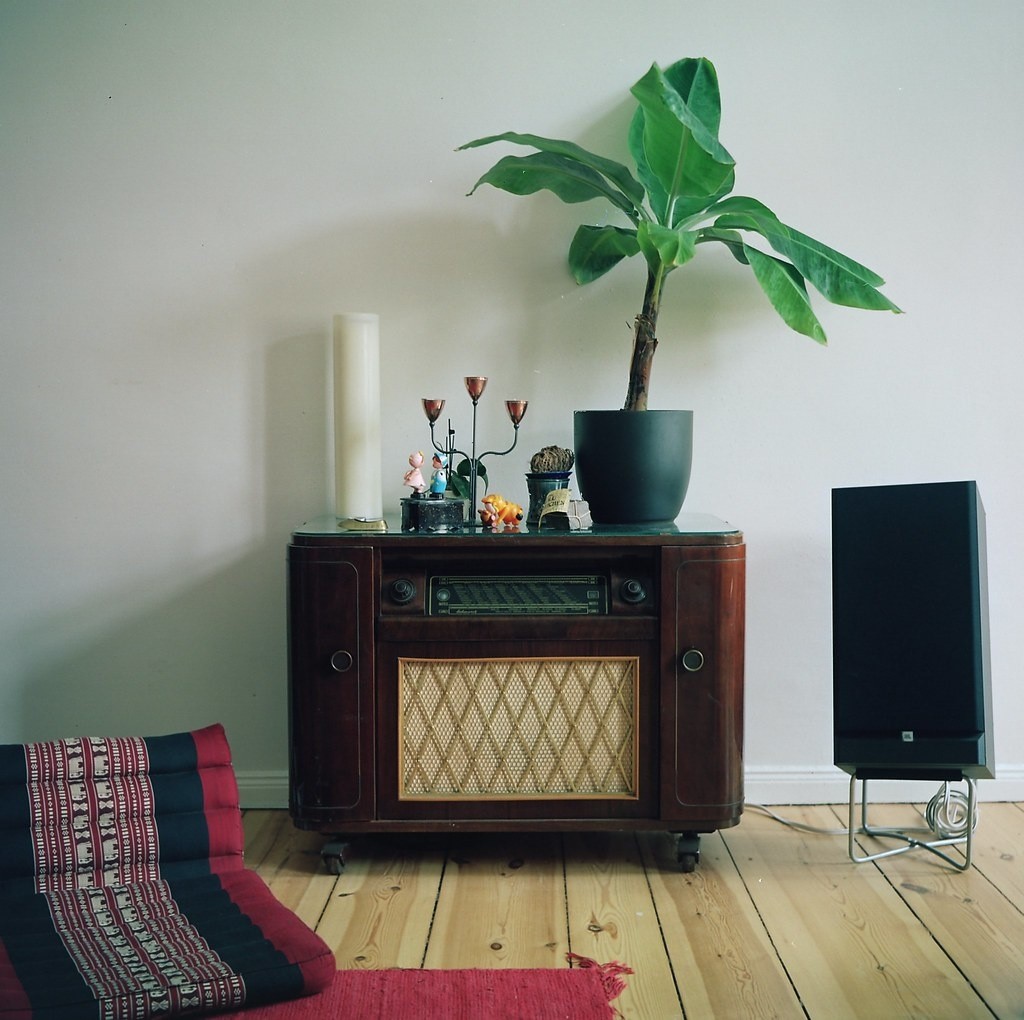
[524, 446, 575, 527]
[453, 57, 907, 523]
[424, 458, 489, 520]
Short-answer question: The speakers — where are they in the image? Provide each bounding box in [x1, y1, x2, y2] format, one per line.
[833, 480, 996, 780]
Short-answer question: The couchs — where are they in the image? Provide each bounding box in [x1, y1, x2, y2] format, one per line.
[0, 723, 335, 1020]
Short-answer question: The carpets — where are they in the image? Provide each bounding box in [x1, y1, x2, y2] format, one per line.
[212, 952, 635, 1020]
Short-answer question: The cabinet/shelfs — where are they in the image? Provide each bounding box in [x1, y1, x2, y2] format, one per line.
[287, 511, 749, 877]
